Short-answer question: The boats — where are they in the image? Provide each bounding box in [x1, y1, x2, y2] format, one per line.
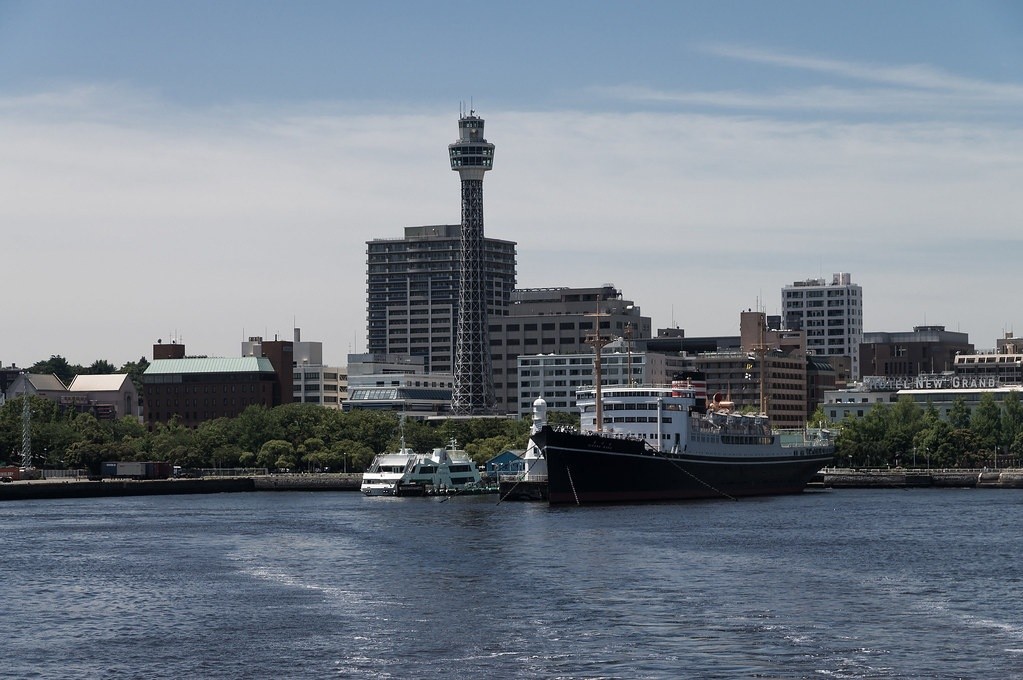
[729, 410, 742, 422]
[711, 410, 728, 423]
[756, 412, 768, 423]
[743, 411, 756, 423]
[422, 483, 500, 495]
[359, 446, 480, 497]
[817, 463, 908, 487]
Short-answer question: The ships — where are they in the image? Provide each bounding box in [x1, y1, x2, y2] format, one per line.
[531, 292, 837, 506]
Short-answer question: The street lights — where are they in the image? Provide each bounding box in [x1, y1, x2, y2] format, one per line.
[914, 446, 916, 467]
[995, 445, 1000, 469]
[927, 448, 929, 475]
